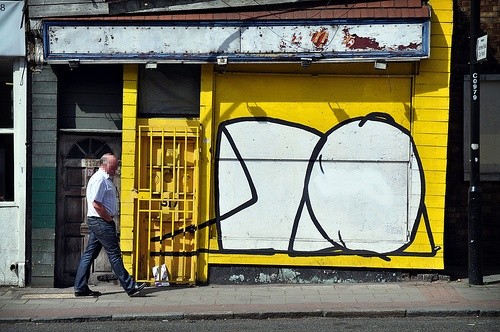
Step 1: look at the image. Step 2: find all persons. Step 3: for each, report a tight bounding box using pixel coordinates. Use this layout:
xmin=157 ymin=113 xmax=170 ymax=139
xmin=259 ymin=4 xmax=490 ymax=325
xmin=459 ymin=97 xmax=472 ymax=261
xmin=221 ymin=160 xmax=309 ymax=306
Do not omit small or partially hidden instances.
xmin=74 ymin=154 xmax=147 ymax=299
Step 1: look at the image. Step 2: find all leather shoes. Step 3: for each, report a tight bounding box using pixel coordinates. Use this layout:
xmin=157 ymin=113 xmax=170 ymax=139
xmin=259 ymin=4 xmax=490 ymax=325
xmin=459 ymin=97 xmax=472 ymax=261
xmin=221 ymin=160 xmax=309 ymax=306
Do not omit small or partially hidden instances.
xmin=75 ymin=287 xmax=102 ymax=297
xmin=129 ymin=281 xmax=147 ymax=297
